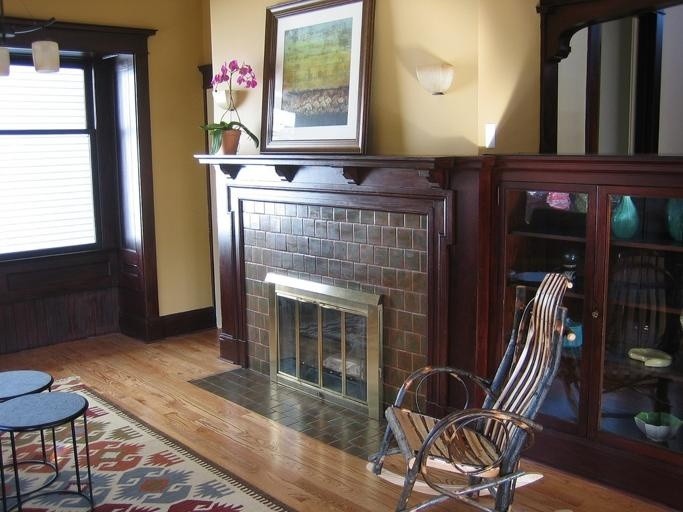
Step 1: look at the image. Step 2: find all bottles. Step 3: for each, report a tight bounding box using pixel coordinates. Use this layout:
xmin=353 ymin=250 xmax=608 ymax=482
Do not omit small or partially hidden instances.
xmin=610 ymin=196 xmax=639 ymax=240
xmin=562 ymin=253 xmax=578 ymax=292
xmin=665 ymin=199 xmax=683 ymax=242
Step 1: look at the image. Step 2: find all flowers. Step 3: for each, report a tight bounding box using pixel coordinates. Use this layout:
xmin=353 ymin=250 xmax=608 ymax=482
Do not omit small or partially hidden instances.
xmin=200 ymin=60 xmax=259 ymax=153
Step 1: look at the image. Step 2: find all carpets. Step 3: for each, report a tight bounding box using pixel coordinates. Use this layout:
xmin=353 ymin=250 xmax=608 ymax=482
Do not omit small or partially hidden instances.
xmin=0 ymin=377 xmax=301 ymax=512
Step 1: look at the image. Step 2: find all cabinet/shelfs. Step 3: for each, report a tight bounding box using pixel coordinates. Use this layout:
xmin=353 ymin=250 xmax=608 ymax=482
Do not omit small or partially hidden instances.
xmin=476 ymin=159 xmax=683 ymax=512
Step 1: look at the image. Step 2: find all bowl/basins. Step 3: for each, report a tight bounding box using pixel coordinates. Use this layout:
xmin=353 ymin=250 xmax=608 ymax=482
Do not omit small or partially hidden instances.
xmin=633 ymin=411 xmax=683 ymax=443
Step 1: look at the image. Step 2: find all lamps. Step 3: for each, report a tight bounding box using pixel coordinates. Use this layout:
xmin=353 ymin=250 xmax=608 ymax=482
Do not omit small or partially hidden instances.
xmin=213 ymin=90 xmax=246 ymax=110
xmin=417 ymin=64 xmax=454 ymax=95
xmin=0 ymin=0 xmax=59 ymax=77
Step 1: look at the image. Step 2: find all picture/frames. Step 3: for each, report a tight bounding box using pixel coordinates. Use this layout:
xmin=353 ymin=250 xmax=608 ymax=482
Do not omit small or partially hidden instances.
xmin=259 ymin=0 xmax=376 ymax=155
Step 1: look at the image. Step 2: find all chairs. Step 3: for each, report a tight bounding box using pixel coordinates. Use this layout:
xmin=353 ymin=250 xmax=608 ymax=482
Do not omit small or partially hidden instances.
xmin=367 ymin=272 xmax=571 ymax=511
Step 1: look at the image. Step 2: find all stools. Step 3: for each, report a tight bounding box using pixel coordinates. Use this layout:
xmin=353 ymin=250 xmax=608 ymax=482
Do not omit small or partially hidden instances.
xmin=0 ymin=369 xmax=59 ymax=499
xmin=0 ymin=392 xmax=93 ymax=512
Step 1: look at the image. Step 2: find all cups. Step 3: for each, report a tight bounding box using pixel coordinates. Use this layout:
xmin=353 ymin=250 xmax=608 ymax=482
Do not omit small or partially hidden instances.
xmin=563 ymin=322 xmax=583 ymax=349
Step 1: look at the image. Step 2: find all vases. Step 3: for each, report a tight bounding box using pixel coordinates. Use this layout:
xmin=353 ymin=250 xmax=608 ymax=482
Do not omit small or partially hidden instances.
xmin=222 ymin=130 xmax=241 ymax=155
